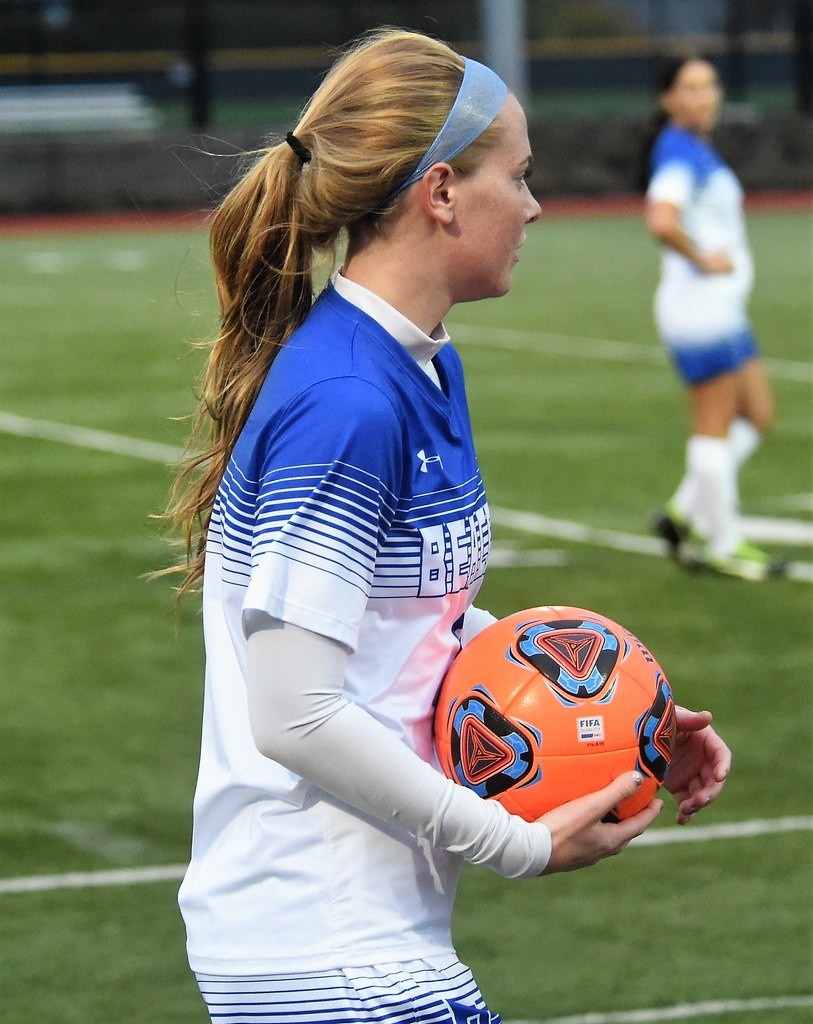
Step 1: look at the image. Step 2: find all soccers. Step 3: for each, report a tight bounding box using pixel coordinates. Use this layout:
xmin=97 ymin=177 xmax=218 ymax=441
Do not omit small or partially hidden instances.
xmin=432 ymin=603 xmax=676 ymax=827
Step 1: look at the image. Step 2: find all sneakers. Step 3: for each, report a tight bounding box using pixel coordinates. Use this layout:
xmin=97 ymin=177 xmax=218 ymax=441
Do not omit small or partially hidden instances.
xmin=657 ymin=502 xmax=785 ymax=583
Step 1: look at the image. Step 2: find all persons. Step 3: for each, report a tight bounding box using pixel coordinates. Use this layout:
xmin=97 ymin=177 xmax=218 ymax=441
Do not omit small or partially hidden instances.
xmin=647 ymin=57 xmax=785 ymax=587
xmin=142 ymin=25 xmax=733 ymax=1023
